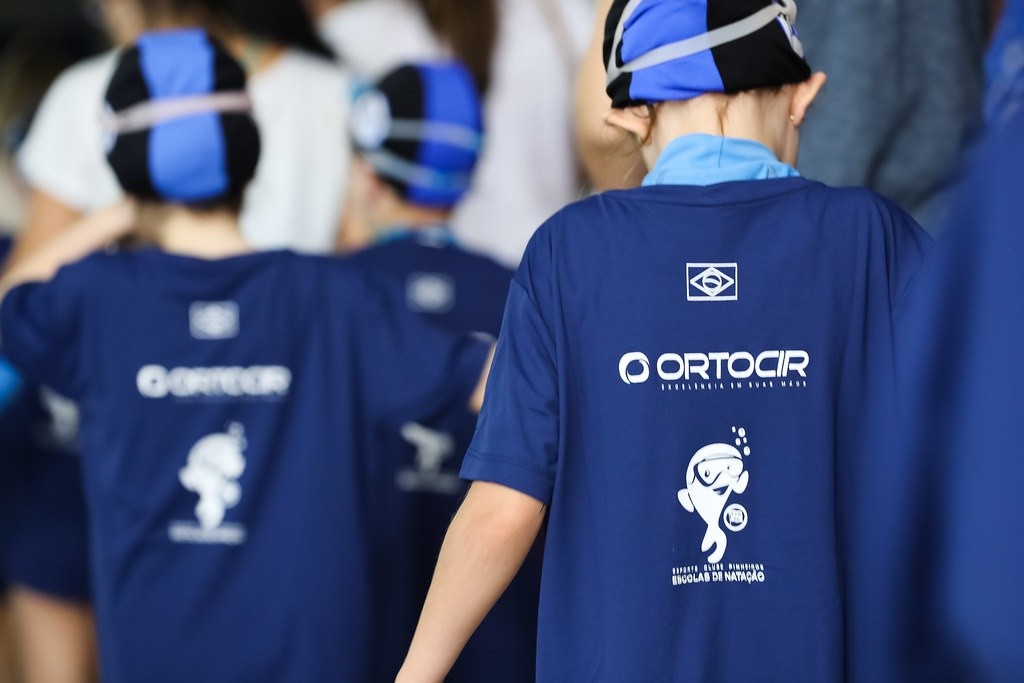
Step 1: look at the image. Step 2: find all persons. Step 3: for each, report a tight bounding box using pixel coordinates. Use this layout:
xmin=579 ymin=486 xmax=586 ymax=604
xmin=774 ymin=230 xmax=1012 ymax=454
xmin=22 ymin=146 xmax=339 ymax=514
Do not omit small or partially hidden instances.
xmin=395 ymin=0 xmax=939 ymax=683
xmin=1 ymin=0 xmax=1024 ymax=683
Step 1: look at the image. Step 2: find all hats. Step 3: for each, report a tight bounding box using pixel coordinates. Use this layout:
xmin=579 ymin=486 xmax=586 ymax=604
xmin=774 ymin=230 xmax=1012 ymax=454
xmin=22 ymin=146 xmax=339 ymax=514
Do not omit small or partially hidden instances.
xmin=602 ymin=0 xmax=811 ymax=109
xmin=106 ymin=29 xmax=262 ymax=203
xmin=346 ymin=62 xmax=484 ymax=206
